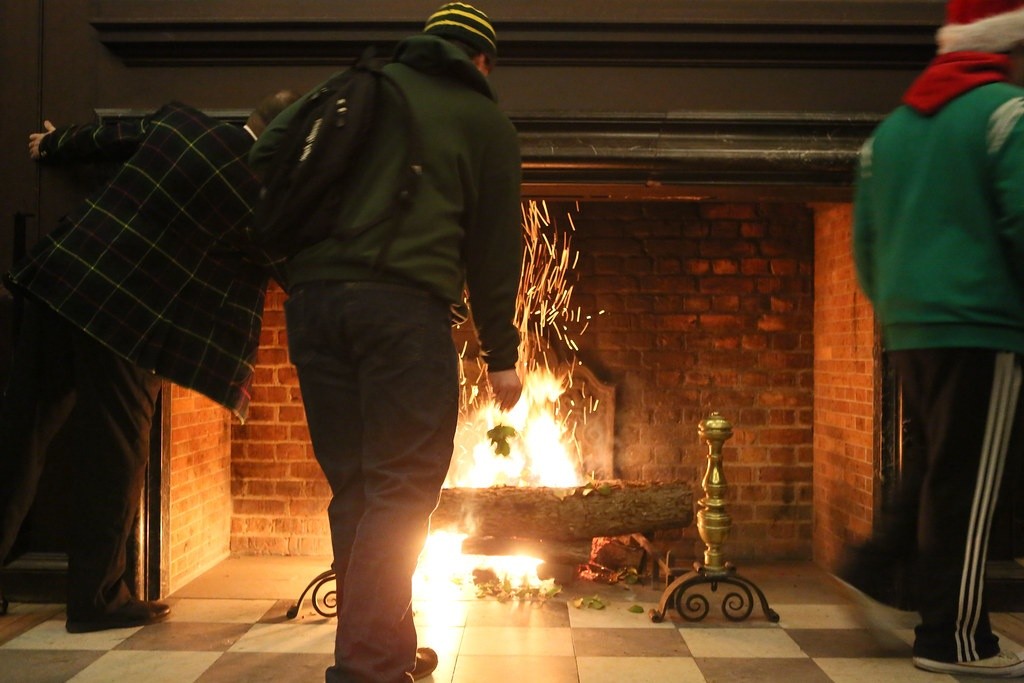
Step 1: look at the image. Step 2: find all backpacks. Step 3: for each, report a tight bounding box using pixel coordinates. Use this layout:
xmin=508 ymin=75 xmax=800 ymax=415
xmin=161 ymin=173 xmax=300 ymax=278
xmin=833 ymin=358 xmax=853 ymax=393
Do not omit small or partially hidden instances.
xmin=243 ymin=59 xmax=422 ymax=258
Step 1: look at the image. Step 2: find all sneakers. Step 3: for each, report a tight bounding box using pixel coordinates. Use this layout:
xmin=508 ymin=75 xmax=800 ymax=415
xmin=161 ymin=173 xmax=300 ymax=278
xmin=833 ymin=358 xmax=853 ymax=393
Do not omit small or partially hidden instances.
xmin=912 ymin=652 xmax=1024 ymax=677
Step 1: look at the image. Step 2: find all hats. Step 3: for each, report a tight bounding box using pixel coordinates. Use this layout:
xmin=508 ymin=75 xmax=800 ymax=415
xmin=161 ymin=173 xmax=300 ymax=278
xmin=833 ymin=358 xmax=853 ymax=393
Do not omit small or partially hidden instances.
xmin=933 ymin=1 xmax=1023 ymax=56
xmin=423 ymin=2 xmax=496 ymax=74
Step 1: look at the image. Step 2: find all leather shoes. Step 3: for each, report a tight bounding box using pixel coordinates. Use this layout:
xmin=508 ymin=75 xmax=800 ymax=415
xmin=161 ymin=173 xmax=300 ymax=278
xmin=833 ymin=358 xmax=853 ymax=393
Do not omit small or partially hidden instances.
xmin=65 ymin=597 xmax=172 ymax=633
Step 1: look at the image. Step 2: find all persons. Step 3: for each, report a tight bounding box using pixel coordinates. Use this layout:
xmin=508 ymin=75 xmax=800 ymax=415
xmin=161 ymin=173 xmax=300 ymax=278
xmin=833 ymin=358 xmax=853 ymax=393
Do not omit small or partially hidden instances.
xmin=828 ymin=0 xmax=1024 ymax=683
xmin=254 ymin=4 xmax=522 ymax=683
xmin=1 ymin=87 xmax=303 ymax=633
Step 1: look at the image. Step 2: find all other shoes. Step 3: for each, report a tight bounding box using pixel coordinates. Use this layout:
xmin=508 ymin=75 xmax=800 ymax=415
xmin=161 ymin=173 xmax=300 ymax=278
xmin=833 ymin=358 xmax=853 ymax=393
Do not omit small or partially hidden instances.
xmin=412 ymin=647 xmax=438 ymax=679
